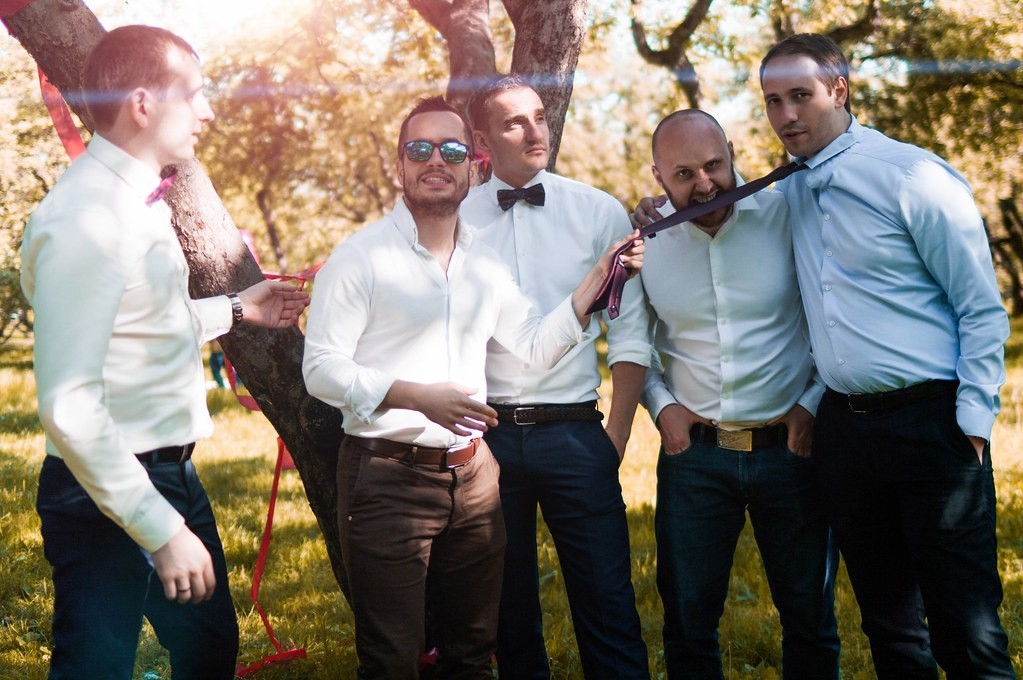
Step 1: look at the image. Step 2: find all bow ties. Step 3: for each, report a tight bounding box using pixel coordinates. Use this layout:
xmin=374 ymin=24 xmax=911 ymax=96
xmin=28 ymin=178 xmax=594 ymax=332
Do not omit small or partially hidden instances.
xmin=497 ymin=182 xmax=546 ymax=212
xmin=146 ymin=174 xmax=177 ymax=205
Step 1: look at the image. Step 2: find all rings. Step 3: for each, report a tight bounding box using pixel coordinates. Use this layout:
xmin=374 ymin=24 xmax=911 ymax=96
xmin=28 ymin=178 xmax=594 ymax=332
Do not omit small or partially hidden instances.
xmin=178 ymin=587 xmax=191 ymax=592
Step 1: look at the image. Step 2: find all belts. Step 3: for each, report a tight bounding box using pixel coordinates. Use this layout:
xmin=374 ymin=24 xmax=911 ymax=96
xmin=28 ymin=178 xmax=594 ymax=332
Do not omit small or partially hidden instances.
xmin=656 ymin=414 xmax=789 ymax=452
xmin=492 ymin=406 xmax=604 ymax=425
xmin=823 ymin=381 xmax=955 ymax=414
xmin=134 ymin=441 xmax=195 ymax=462
xmin=345 ymin=433 xmax=480 ymax=469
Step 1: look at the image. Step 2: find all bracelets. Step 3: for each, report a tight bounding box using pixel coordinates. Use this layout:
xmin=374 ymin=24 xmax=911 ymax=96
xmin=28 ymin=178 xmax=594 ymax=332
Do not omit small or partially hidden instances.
xmin=226 ymin=292 xmax=243 ymax=326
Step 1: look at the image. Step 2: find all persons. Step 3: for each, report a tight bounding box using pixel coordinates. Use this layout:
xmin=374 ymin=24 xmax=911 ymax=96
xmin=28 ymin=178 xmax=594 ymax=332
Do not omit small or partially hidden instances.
xmin=303 ymin=96 xmax=645 ymax=680
xmin=631 ymin=33 xmax=1015 ymax=680
xmin=20 ymin=25 xmax=312 ymax=680
xmin=459 ymin=75 xmax=651 ymax=680
xmin=638 ymin=109 xmax=841 ymax=680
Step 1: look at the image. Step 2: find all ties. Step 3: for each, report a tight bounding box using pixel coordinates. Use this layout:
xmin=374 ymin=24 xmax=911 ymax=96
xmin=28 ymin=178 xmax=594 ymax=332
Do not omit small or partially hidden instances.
xmin=584 ymin=156 xmax=810 ymax=321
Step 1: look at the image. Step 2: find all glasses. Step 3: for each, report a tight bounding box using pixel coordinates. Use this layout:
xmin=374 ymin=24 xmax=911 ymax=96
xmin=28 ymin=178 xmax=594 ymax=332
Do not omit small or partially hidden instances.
xmin=399 ymin=140 xmax=473 ymax=164
